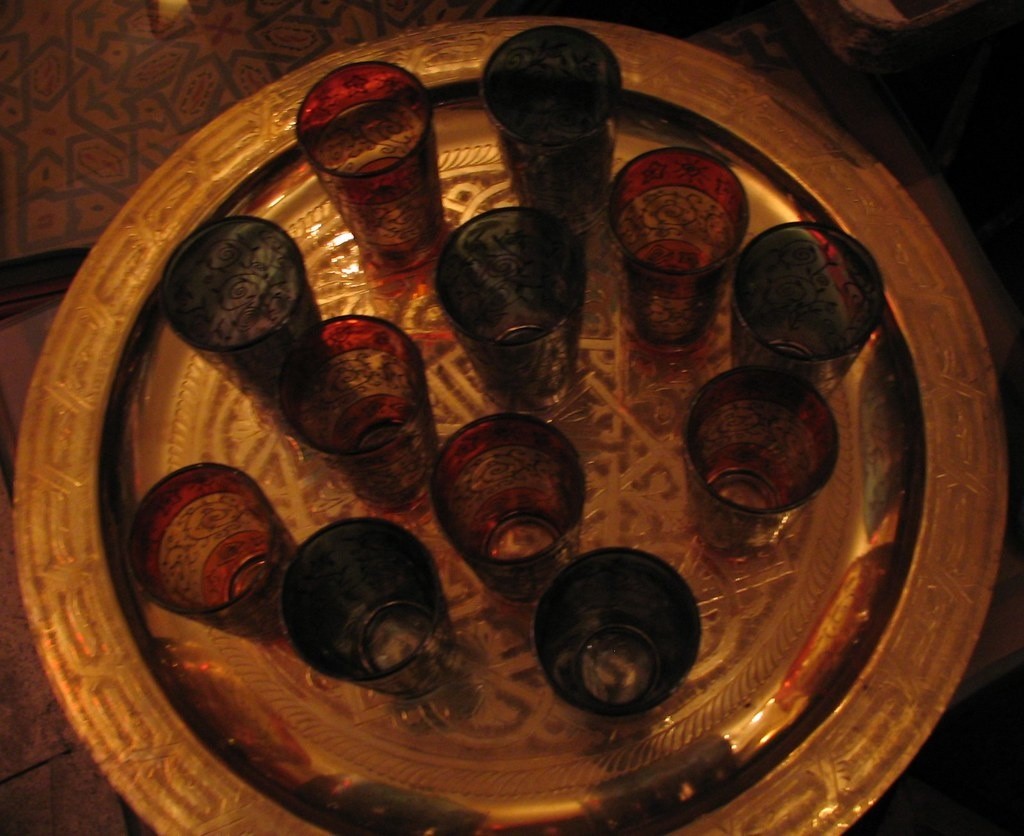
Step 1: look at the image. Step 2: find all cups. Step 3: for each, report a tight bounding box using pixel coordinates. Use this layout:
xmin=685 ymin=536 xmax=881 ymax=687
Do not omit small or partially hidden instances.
xmin=123 ymin=24 xmax=886 ymax=717
